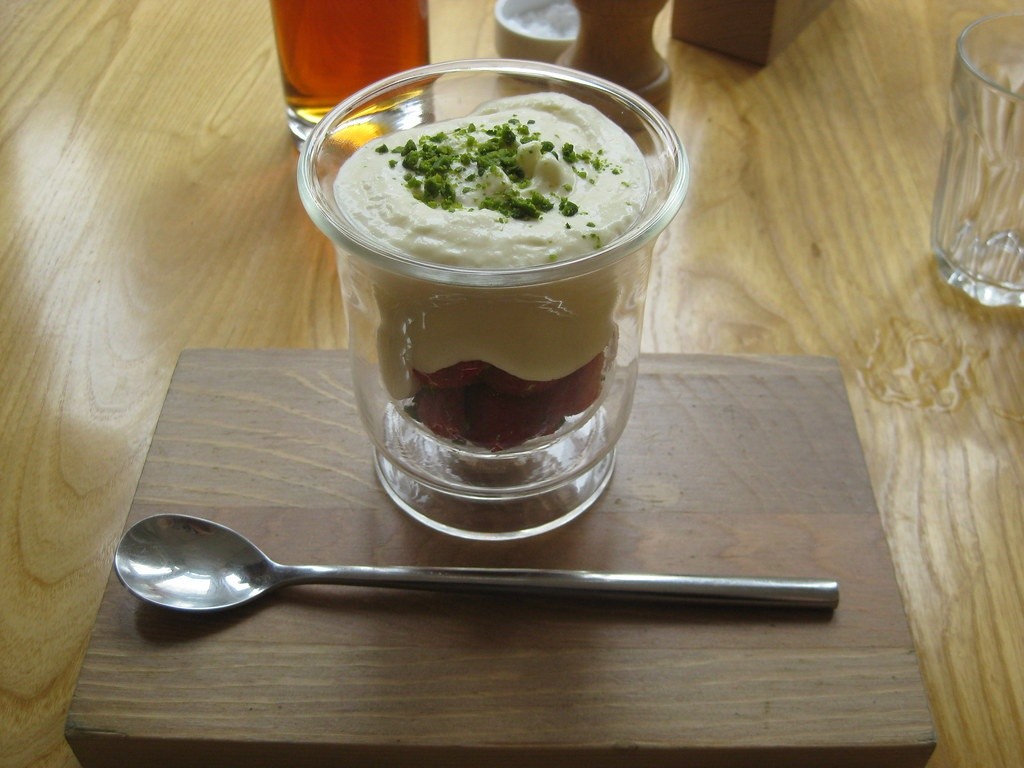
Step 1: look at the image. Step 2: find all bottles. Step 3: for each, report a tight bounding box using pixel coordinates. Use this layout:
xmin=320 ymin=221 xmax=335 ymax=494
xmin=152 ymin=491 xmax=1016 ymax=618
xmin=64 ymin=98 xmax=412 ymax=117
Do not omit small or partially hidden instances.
xmin=546 ymin=0 xmax=671 ymax=111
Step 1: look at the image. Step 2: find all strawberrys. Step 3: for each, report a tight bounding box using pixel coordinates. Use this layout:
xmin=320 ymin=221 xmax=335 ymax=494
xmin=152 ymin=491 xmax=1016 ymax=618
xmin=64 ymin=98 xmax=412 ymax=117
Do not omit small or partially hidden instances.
xmin=408 ymin=344 xmax=607 ymax=452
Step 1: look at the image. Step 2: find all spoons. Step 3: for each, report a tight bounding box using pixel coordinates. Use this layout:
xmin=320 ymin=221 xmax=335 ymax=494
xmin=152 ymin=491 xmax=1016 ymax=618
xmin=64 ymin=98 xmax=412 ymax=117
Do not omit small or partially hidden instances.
xmin=113 ymin=515 xmax=841 ymax=610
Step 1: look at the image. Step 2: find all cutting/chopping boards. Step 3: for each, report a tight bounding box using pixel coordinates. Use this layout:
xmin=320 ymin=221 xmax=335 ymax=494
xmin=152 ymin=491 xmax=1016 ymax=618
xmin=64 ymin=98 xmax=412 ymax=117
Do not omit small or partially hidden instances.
xmin=64 ymin=347 xmax=939 ymax=767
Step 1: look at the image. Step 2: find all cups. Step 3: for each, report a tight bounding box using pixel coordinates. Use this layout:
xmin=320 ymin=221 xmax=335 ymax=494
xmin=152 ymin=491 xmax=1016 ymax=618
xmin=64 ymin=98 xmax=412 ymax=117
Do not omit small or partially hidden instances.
xmin=929 ymin=10 xmax=1024 ymax=308
xmin=298 ymin=60 xmax=689 ymax=542
xmin=271 ymin=0 xmax=430 ymax=145
xmin=495 ymin=0 xmax=580 ymax=64
